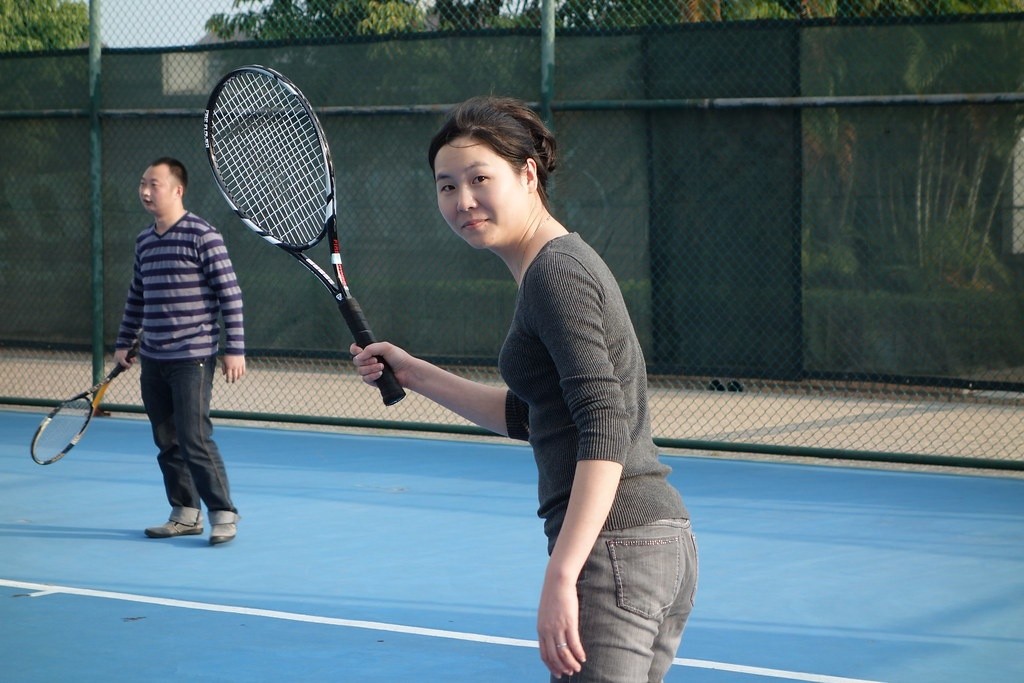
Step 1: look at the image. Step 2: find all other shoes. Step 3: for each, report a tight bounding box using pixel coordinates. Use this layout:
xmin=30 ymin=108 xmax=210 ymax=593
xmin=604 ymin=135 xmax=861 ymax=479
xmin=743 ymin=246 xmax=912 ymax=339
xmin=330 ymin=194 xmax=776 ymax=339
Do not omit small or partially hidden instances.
xmin=144 ymin=520 xmax=204 ymax=537
xmin=209 ymin=522 xmax=237 ymax=543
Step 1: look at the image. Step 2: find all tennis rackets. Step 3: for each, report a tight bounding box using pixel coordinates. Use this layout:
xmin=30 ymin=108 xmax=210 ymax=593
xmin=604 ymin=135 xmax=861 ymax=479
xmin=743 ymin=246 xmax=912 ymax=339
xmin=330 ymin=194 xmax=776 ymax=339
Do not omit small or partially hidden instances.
xmin=200 ymin=62 xmax=408 ymax=409
xmin=30 ymin=341 xmax=140 ymax=466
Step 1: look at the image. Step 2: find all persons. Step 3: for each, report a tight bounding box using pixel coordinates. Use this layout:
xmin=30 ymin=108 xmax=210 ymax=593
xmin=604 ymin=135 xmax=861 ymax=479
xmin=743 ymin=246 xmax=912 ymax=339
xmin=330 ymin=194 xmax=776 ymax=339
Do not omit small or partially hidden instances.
xmin=349 ymin=96 xmax=698 ymax=683
xmin=113 ymin=158 xmax=246 ymax=544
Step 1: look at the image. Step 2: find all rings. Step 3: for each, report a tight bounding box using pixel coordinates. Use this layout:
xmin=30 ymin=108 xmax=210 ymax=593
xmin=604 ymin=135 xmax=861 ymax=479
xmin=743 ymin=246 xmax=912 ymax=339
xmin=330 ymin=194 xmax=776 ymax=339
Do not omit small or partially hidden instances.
xmin=556 ymin=643 xmax=568 ymax=647
